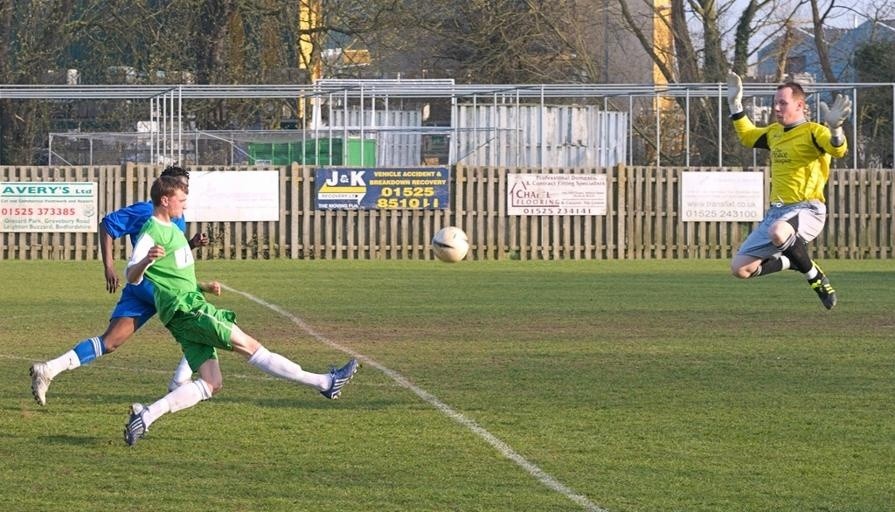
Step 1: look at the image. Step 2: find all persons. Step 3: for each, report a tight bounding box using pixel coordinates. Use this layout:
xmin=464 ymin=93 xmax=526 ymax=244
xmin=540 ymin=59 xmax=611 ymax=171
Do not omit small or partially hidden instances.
xmin=725 ymin=68 xmax=853 ymax=313
xmin=121 ymin=175 xmax=361 ymax=446
xmin=28 ymin=166 xmax=210 ymax=408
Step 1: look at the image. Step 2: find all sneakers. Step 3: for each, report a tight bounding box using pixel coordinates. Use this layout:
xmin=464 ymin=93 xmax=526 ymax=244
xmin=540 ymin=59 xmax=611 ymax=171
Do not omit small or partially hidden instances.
xmin=122 ymin=401 xmax=154 ymax=446
xmin=804 ymin=258 xmax=839 ymax=310
xmin=167 ymin=377 xmax=195 ymax=392
xmin=319 ymin=357 xmax=361 ymax=400
xmin=29 ymin=360 xmax=55 ymax=409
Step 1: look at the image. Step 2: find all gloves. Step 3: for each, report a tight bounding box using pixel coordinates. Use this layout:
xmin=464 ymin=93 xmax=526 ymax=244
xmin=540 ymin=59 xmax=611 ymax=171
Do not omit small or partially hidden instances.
xmin=725 ymin=68 xmax=746 ymax=116
xmin=819 ymin=92 xmax=854 ymax=137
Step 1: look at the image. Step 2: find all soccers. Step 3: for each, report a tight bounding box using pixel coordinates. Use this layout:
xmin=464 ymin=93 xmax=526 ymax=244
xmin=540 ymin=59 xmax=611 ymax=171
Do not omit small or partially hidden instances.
xmin=432 ymin=227 xmax=468 ymax=263
